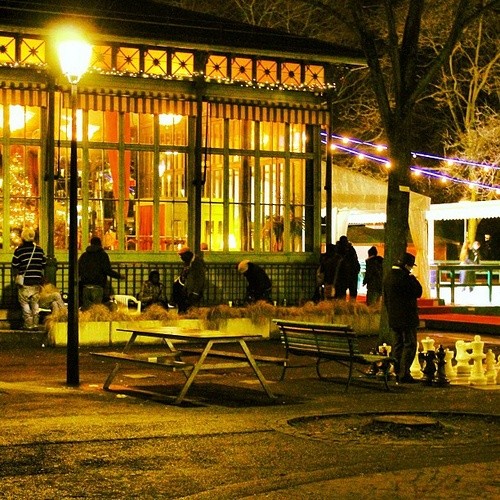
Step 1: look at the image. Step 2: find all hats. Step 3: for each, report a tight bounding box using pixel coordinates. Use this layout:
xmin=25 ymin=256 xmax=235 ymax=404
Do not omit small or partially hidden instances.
xmin=398 ymin=252 xmax=418 ymax=267
xmin=368 ymin=246 xmax=378 ymax=254
xmin=237 ymin=259 xmax=250 ymax=273
xmin=178 ymin=247 xmax=192 ymax=255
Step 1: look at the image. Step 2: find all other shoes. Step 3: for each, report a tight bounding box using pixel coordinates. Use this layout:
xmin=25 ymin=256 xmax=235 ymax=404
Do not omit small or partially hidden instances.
xmin=19 ymin=324 xmax=39 ymax=330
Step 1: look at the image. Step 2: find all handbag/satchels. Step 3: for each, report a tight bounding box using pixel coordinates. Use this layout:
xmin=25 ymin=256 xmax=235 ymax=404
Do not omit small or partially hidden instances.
xmin=15 ymin=274 xmax=24 ymax=286
xmin=324 ymin=282 xmax=335 ymax=298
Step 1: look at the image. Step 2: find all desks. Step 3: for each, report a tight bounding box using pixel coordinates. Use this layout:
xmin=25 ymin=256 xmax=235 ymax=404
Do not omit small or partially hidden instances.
xmin=436 ymin=264 xmax=500 ymax=304
xmin=87 ymin=328 xmax=291 ymax=407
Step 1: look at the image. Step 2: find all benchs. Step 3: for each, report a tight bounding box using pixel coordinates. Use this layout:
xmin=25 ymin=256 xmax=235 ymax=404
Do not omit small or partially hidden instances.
xmin=273 ymin=316 xmax=393 ymax=392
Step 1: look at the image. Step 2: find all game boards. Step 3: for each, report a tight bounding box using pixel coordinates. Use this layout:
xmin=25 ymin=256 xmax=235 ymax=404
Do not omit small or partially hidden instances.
xmin=364 ymin=363 xmax=500 ymax=391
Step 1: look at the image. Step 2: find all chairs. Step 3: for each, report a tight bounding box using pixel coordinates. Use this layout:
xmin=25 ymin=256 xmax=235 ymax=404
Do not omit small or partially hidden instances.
xmin=107 ymin=294 xmax=142 ymax=316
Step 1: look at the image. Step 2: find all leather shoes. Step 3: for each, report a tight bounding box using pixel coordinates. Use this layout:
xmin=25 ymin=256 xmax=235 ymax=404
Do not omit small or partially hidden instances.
xmin=396 ymin=372 xmax=421 ymax=384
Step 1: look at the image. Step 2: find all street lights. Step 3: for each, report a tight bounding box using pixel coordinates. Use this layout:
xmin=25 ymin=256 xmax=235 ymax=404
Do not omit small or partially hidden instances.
xmin=55 ymin=18 xmax=97 ymax=390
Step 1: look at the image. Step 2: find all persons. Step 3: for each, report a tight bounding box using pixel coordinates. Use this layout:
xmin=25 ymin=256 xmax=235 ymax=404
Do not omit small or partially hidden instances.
xmin=167 ymin=248 xmax=206 ymax=315
xmin=383 ymin=252 xmax=423 ymax=384
xmin=310 ymin=235 xmax=362 ymax=303
xmin=481 ymin=233 xmax=498 ymax=280
xmin=238 ymin=260 xmax=275 ymax=306
xmin=362 ymin=246 xmax=384 ymax=306
xmin=138 ymin=271 xmax=169 ymax=313
xmin=77 ymin=236 xmax=125 ymax=312
xmin=462 ymin=241 xmax=480 ymax=292
xmin=11 ymin=227 xmax=49 ymax=331
